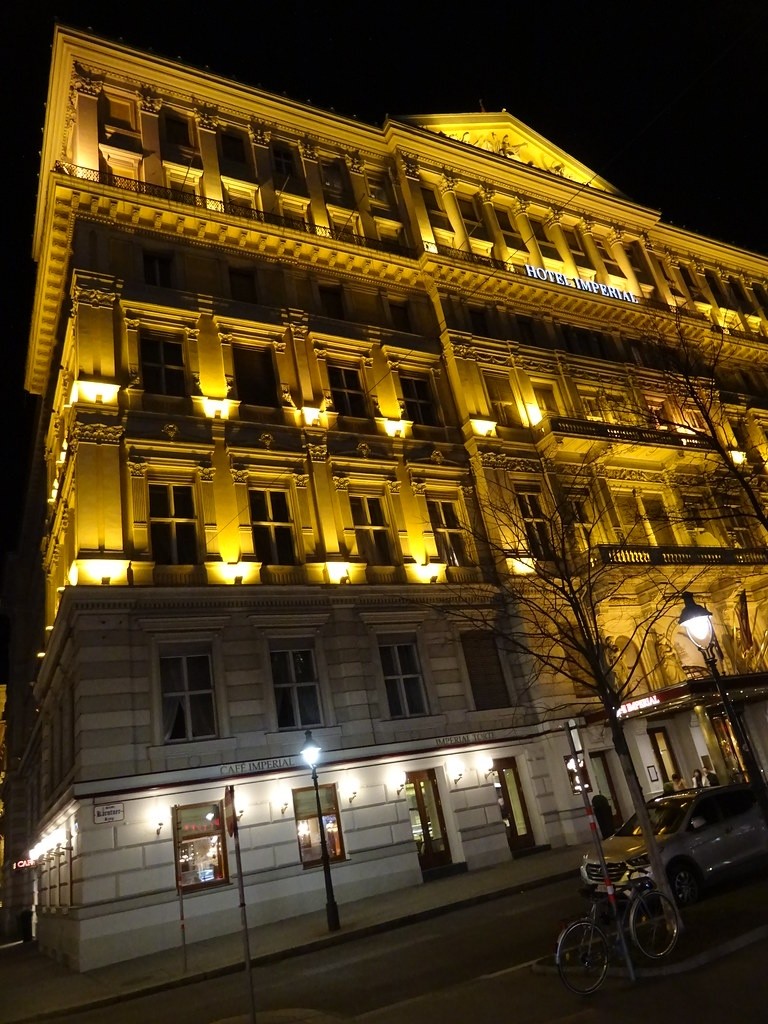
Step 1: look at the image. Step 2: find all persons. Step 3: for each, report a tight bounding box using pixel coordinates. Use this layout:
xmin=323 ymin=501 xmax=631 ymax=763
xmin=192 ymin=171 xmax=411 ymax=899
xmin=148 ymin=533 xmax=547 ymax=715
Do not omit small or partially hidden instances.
xmin=664 ymin=767 xmax=720 ymax=795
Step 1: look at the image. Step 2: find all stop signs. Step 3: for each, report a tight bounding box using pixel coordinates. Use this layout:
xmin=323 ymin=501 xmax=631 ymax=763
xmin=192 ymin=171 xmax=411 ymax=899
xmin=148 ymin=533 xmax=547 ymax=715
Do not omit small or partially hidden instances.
xmin=225 ymin=787 xmax=236 ymax=838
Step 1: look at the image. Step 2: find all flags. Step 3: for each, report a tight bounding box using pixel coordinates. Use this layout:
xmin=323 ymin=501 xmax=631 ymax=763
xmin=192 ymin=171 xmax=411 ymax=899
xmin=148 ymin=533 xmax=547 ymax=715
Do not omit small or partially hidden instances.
xmin=735 ymin=589 xmax=754 ymax=650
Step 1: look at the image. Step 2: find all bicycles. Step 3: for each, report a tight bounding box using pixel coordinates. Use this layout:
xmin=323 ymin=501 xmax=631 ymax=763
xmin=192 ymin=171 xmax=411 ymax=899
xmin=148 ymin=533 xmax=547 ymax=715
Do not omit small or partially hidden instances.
xmin=553 ymin=867 xmax=680 ymax=995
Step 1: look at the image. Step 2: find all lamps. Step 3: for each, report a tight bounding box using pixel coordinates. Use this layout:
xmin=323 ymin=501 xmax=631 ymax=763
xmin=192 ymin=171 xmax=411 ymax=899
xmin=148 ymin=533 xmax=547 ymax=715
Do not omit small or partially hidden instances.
xmin=29 ymin=829 xmax=73 ymax=868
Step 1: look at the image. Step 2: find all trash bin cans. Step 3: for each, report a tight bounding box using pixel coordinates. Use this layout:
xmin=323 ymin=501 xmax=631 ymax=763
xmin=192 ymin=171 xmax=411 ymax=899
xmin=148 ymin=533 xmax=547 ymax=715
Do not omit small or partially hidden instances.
xmin=16 ymin=910 xmax=33 ymax=943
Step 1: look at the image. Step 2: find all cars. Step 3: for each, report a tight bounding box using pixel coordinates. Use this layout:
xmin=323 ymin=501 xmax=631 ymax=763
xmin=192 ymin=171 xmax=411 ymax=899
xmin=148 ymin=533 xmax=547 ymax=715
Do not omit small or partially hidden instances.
xmin=581 ymin=783 xmax=767 ymax=906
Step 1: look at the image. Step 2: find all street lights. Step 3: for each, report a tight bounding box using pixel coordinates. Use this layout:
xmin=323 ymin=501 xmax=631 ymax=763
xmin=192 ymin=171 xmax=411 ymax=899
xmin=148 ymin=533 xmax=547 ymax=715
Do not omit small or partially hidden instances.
xmin=679 ymin=589 xmax=768 ymax=820
xmin=299 ymin=729 xmax=340 ymax=931
